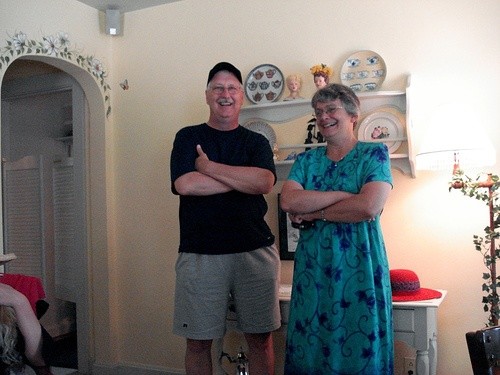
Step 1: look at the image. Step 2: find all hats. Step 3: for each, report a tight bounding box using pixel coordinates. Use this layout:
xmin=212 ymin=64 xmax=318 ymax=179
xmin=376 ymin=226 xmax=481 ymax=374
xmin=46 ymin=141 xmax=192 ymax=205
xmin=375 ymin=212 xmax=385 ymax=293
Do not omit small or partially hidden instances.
xmin=207 ymin=62 xmax=242 ymax=83
xmin=390 ymin=269 xmax=442 ymax=302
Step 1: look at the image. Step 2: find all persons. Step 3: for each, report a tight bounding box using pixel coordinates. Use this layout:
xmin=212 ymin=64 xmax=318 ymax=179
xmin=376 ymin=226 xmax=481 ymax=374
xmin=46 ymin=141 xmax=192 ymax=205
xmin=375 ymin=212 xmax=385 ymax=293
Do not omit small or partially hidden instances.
xmin=310 ymin=64 xmax=333 ymax=91
xmin=168 ymin=62 xmax=283 ymax=375
xmin=277 ymin=83 xmax=395 ymax=375
xmin=283 ymin=74 xmax=306 ymax=101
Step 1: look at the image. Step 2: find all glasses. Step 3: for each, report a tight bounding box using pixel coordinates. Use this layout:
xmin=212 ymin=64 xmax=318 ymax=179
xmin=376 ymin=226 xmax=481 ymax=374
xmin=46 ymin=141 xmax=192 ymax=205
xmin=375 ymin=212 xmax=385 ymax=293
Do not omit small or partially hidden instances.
xmin=312 ymin=105 xmax=345 ymax=117
xmin=207 ymin=85 xmax=242 ymax=94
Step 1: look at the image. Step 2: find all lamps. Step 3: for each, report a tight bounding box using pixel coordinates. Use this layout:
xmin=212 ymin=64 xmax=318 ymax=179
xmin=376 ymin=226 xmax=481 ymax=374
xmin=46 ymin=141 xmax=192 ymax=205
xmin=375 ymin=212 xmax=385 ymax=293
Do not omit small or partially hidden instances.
xmin=104 ymin=7 xmax=120 ymax=35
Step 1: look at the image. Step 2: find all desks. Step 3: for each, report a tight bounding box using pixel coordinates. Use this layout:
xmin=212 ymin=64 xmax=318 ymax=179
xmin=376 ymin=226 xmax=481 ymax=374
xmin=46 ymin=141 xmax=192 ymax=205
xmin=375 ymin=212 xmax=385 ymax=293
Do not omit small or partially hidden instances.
xmin=231 ymin=284 xmax=447 ymax=375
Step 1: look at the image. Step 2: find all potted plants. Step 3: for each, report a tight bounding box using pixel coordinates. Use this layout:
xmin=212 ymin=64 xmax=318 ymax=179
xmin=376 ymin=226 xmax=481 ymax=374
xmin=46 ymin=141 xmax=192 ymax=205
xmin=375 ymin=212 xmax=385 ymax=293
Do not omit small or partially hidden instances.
xmin=447 ymin=162 xmax=500 ymax=375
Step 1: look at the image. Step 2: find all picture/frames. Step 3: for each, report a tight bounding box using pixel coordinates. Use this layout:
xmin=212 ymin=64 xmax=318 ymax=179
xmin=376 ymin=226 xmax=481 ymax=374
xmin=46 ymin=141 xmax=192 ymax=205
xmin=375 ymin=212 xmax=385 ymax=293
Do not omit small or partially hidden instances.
xmin=276 ymin=193 xmax=300 ymax=262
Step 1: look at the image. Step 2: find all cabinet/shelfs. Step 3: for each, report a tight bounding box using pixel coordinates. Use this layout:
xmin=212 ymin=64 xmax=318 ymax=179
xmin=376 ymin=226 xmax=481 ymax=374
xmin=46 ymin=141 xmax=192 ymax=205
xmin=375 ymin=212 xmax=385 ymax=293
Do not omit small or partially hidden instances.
xmin=240 ymin=91 xmax=408 ymax=167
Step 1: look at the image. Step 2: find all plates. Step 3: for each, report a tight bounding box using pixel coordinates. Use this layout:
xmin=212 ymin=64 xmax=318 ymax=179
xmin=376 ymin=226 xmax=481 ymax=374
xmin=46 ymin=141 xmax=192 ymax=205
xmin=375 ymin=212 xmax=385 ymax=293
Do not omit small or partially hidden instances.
xmin=242 ymin=120 xmax=276 ymax=152
xmin=243 ymin=63 xmax=285 ymax=104
xmin=357 ymin=111 xmax=404 ymax=154
xmin=339 ymin=50 xmax=387 ymax=93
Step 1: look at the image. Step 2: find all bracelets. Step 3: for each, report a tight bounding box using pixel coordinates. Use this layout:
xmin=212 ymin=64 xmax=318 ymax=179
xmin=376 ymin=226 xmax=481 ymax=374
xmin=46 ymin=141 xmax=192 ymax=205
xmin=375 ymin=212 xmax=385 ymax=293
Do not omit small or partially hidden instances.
xmin=320 ymin=209 xmax=326 ymax=222
